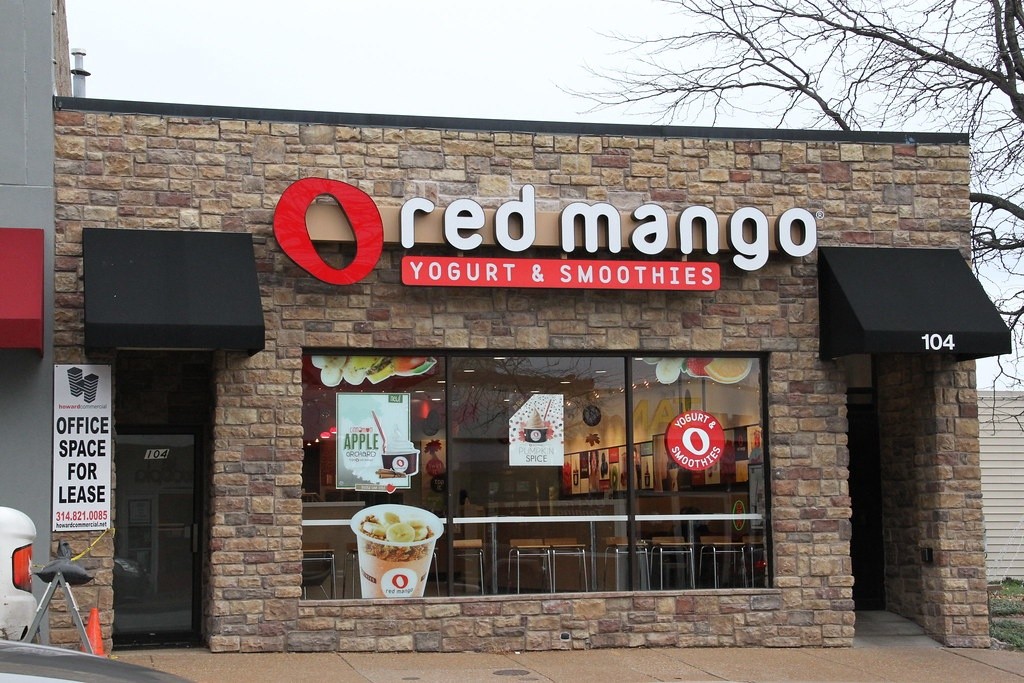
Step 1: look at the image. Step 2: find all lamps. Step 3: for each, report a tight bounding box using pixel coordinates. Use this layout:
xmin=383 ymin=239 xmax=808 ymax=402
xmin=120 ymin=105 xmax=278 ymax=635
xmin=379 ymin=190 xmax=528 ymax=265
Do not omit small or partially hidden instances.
xmin=320 ymin=431 xmax=331 ymax=439
xmin=328 ymin=426 xmax=336 ymax=434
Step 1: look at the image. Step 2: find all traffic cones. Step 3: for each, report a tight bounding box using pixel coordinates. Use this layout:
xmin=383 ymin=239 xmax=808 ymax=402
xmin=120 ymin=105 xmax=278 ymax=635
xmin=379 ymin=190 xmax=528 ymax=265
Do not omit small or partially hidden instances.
xmin=80 ymin=608 xmax=104 ymax=655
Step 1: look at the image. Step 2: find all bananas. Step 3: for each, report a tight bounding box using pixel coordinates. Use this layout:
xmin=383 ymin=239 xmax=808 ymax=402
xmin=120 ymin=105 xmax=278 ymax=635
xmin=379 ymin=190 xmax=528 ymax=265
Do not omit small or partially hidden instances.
xmin=363 ymin=513 xmax=429 ymax=543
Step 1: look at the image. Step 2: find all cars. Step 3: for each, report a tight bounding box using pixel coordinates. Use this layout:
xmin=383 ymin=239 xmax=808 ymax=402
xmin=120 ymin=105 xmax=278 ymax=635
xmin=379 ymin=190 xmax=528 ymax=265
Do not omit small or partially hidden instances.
xmin=1 ymin=506 xmax=39 ymax=642
xmin=0 ymin=638 xmax=196 ymax=683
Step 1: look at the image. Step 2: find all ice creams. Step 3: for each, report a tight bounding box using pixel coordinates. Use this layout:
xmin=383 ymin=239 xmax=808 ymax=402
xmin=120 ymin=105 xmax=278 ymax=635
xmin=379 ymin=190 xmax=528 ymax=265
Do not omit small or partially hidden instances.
xmin=523 ymin=409 xmax=548 ymax=443
xmin=381 ymin=430 xmax=420 ymax=477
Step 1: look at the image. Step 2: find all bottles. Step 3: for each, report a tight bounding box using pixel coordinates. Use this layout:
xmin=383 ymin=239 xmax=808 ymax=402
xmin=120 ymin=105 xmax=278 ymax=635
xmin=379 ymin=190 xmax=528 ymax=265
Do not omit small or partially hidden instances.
xmin=644 ymin=463 xmax=650 ymax=487
xmin=572 ymin=460 xmax=579 ymax=486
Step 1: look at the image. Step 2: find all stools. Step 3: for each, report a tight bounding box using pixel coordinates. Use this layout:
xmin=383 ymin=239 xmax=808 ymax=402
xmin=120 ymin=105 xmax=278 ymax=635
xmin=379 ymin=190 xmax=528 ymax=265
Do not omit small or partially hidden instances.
xmin=298 ymin=536 xmax=765 ymax=601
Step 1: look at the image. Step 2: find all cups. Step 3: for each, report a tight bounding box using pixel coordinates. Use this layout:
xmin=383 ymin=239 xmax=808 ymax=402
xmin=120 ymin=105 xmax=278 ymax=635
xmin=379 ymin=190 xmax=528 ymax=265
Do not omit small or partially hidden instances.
xmin=350 ymin=504 xmax=444 ymax=598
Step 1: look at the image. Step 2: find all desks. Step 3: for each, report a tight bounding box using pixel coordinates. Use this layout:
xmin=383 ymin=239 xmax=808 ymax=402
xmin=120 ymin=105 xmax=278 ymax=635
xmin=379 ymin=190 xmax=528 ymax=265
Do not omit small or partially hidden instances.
xmin=300 ymin=514 xmax=763 ymax=596
